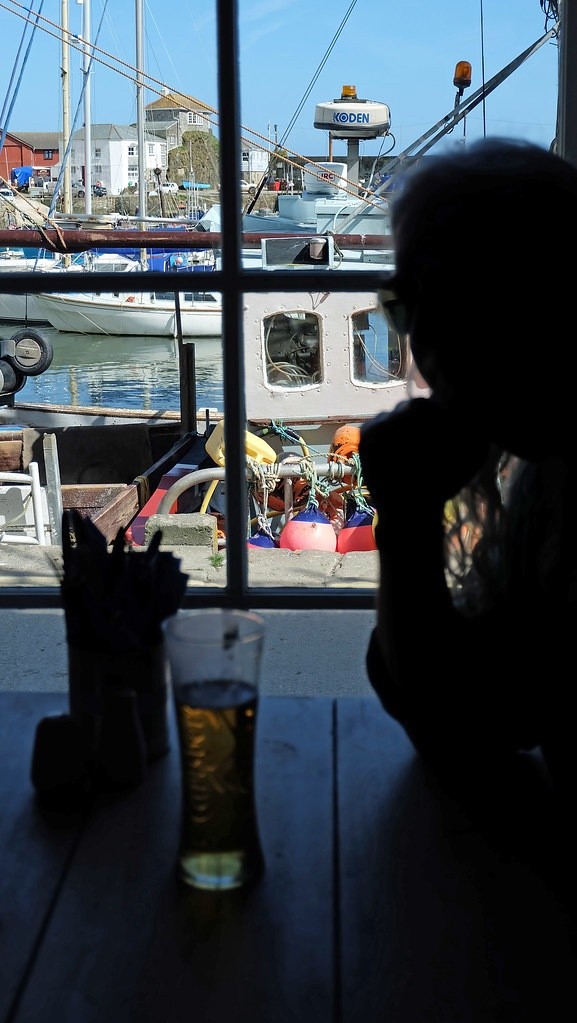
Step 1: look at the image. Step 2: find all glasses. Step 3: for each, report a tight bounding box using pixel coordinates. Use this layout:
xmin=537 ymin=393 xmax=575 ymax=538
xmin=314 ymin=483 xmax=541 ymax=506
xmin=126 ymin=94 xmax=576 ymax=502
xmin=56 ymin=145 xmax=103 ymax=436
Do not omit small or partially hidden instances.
xmin=377 ymin=275 xmax=408 ymax=334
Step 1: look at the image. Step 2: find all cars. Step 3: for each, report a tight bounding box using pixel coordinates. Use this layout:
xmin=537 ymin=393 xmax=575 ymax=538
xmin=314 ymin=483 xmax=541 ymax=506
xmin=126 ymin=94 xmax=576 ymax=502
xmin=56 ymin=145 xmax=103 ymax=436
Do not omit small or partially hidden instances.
xmin=91 ymin=185 xmax=107 ymax=197
xmin=0 ymin=189 xmax=14 ymax=200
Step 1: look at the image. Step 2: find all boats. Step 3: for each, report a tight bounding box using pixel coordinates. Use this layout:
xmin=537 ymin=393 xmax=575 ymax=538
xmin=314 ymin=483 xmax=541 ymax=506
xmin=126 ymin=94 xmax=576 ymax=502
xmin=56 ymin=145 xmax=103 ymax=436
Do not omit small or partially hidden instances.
xmin=182 ymin=179 xmax=210 ymax=189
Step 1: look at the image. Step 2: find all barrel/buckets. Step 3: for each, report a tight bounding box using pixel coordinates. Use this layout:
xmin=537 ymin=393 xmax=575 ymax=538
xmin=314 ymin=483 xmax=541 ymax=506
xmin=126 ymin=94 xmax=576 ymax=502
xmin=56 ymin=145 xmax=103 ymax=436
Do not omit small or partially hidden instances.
xmin=205 ymin=420 xmax=277 ymax=467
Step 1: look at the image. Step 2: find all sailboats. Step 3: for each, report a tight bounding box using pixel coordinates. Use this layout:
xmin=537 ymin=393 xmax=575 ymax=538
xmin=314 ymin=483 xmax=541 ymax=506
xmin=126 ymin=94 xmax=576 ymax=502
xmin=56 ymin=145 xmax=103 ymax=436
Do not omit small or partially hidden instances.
xmin=0 ymin=0 xmax=561 ymax=338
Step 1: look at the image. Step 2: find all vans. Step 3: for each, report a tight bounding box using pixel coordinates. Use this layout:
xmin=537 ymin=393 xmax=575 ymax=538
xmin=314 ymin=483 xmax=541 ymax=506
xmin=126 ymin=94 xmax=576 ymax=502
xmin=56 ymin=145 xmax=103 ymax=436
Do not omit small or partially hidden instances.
xmin=158 ymin=182 xmax=179 ymax=195
xmin=49 ymin=181 xmax=85 ymax=197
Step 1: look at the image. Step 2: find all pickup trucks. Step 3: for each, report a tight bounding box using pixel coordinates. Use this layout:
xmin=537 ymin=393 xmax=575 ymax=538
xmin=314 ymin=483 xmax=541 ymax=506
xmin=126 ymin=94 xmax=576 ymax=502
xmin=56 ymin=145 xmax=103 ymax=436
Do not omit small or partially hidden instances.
xmin=217 ymin=179 xmax=257 ymax=194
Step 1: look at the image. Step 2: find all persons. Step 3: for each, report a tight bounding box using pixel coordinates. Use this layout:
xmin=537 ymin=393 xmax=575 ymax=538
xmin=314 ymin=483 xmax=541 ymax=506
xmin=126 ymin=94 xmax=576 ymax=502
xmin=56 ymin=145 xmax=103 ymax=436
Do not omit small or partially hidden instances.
xmin=359 ymin=139 xmax=577 ymax=874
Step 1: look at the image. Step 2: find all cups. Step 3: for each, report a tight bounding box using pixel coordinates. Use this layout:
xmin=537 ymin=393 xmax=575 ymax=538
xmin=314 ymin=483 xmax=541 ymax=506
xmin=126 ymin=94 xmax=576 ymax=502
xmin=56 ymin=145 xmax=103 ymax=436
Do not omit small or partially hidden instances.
xmin=162 ymin=611 xmax=269 ymax=891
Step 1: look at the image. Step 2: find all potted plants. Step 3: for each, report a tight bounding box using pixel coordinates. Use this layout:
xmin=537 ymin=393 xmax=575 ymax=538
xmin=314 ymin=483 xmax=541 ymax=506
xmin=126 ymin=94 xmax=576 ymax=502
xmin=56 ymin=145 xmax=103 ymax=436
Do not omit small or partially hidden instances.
xmin=203 ymin=552 xmax=228 ymax=581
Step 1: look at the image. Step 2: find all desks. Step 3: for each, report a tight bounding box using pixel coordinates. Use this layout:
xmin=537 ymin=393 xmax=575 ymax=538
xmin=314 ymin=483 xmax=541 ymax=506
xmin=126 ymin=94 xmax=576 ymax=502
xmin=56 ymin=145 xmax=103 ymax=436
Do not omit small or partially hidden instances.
xmin=1 ymin=682 xmax=513 ymax=1023
